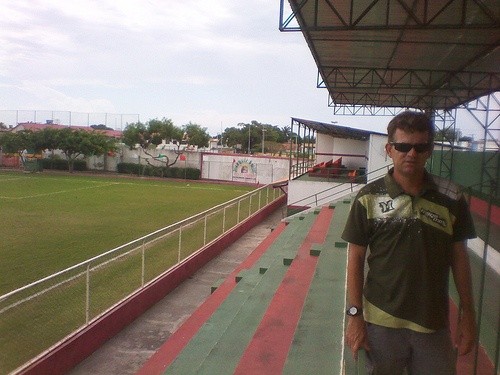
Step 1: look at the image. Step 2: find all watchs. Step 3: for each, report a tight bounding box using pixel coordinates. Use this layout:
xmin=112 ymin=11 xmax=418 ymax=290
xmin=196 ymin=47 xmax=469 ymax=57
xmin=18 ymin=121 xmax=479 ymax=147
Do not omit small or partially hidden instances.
xmin=347 ymin=306 xmax=363 ymax=316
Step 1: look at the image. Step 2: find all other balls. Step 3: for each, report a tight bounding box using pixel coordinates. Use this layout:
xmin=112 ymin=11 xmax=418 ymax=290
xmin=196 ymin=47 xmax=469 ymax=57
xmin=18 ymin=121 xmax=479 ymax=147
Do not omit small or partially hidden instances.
xmin=180 ymin=155 xmax=186 ymax=160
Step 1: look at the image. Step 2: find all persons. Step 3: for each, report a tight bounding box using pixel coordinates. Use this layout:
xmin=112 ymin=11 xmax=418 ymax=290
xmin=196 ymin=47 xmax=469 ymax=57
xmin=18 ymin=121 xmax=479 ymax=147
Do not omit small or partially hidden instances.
xmin=342 ymin=111 xmax=479 ymax=375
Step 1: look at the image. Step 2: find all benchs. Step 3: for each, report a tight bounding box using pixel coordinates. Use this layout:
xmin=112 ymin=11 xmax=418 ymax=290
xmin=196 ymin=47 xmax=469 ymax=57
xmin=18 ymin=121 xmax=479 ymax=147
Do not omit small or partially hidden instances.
xmin=308 ymin=156 xmax=342 ymax=173
